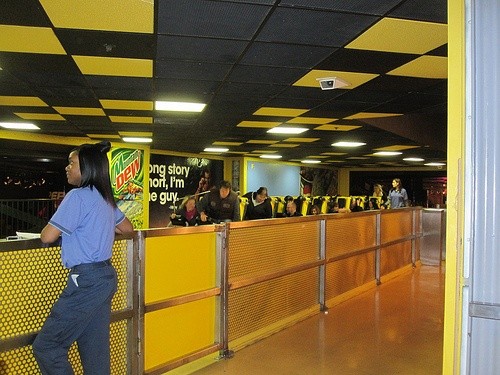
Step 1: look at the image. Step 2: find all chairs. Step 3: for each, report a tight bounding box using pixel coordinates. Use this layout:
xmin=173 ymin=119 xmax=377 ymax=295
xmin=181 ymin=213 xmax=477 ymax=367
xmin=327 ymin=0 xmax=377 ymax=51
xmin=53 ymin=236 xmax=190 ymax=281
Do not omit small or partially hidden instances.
xmin=167 ymin=193 xmax=419 ymax=227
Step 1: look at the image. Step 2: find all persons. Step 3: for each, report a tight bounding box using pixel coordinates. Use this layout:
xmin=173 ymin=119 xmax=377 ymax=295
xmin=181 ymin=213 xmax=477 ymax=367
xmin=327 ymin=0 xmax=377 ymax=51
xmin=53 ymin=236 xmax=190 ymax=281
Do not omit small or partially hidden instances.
xmin=32 ymin=143 xmax=133 ymax=375
xmin=168 ymin=177 xmax=409 ymax=226
xmin=196 ymin=168 xmax=215 ymax=194
xmin=34 ymin=206 xmax=52 ymax=220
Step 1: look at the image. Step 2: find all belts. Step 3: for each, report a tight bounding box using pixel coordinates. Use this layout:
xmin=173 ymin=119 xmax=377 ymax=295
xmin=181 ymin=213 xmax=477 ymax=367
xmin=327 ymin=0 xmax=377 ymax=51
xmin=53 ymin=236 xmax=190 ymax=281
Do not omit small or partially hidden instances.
xmin=70 ymin=260 xmax=112 ymax=272
